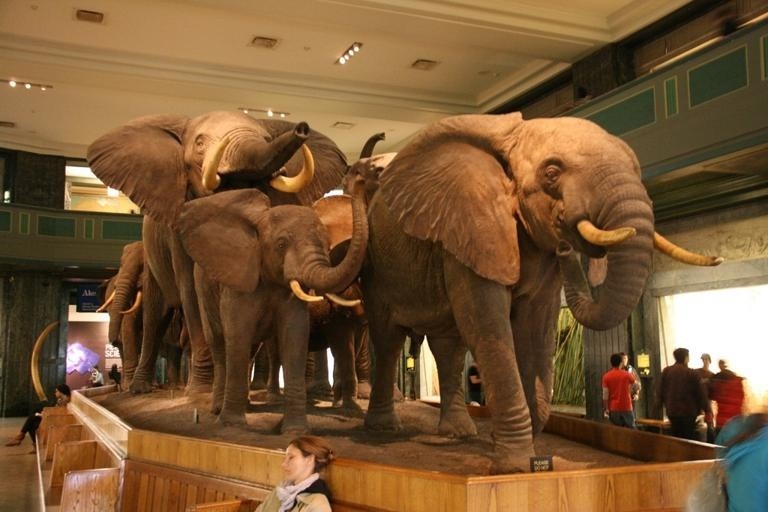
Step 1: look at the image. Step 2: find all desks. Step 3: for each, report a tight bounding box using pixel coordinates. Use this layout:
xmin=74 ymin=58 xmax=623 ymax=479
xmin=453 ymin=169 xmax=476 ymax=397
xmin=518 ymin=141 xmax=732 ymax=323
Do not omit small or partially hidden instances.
xmin=634 ymin=418 xmax=673 ymax=429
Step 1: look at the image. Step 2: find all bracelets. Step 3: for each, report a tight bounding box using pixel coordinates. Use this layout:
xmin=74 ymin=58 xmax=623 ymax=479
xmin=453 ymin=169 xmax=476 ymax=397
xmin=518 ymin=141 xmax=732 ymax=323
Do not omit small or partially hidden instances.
xmin=602 ymin=408 xmax=607 ymax=413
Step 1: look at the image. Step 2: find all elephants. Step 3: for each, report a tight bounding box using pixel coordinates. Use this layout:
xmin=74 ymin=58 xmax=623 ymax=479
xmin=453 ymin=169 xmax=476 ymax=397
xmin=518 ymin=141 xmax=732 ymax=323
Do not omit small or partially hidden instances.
xmin=95 ymin=237 xmax=184 ymax=393
xmin=175 ymin=159 xmax=374 ymax=436
xmin=84 ymin=110 xmax=350 ymax=394
xmin=362 ymin=109 xmax=724 ymax=476
xmin=300 ymin=126 xmax=419 ymax=405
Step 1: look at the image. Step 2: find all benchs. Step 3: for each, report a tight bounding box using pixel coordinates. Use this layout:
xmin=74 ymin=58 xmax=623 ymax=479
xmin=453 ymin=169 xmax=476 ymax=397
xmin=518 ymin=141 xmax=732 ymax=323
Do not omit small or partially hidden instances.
xmin=36 ymin=406 xmax=118 ymax=511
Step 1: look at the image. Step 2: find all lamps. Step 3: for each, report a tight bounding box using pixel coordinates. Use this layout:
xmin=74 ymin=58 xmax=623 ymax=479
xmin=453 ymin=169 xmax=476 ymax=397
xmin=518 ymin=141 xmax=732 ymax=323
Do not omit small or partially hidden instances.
xmin=238 ymin=107 xmax=291 ymax=119
xmin=405 ymin=357 xmax=416 ymax=371
xmin=1 ymin=78 xmax=53 ymax=94
xmin=637 ymin=351 xmax=651 ymax=376
xmin=334 ymin=41 xmax=362 ymax=66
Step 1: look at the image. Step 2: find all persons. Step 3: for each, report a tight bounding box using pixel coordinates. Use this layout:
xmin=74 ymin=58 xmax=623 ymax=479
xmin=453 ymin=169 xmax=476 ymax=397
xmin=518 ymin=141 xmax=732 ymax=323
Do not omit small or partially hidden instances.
xmin=695 ymin=354 xmax=715 ymax=442
xmin=708 ymin=360 xmax=745 ymax=440
xmin=601 ymin=354 xmax=638 ymax=429
xmin=655 ymin=348 xmax=714 ymax=444
xmin=4 ymin=383 xmax=71 ymax=453
xmin=467 ymin=361 xmax=484 ymax=406
xmin=620 ymin=351 xmax=641 ymax=422
xmin=256 ymin=435 xmax=333 ymax=512
xmin=89 ymin=365 xmax=103 ymax=385
xmin=107 ymin=363 xmax=121 ymax=384
